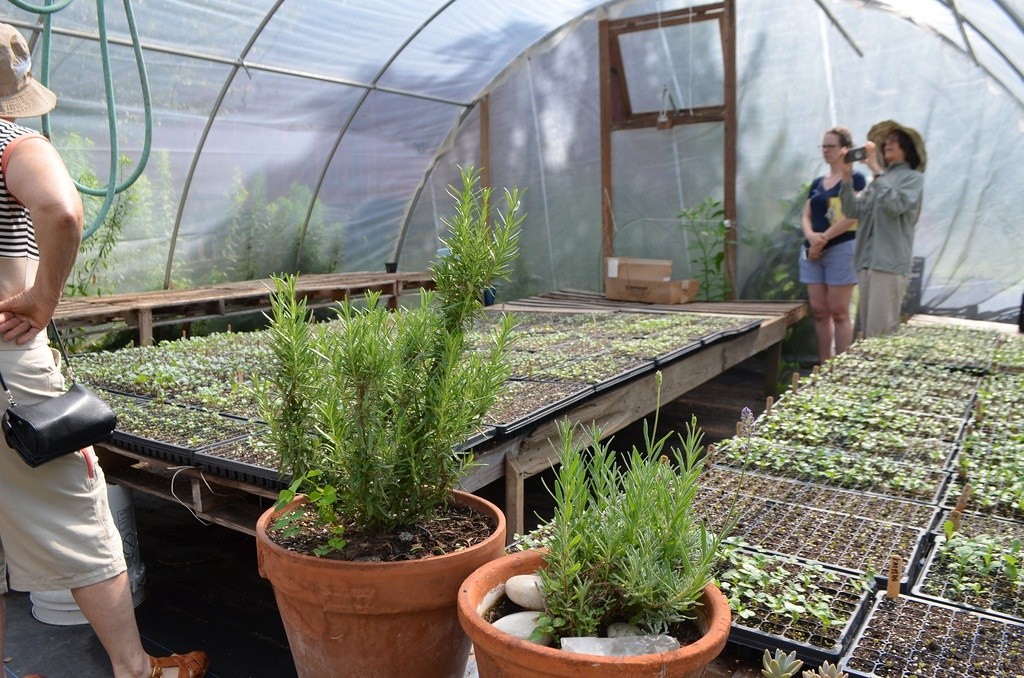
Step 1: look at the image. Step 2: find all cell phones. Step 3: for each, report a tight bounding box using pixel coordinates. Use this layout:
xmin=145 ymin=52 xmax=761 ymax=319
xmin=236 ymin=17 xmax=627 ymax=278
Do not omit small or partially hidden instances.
xmin=845 ymin=147 xmax=868 ymax=163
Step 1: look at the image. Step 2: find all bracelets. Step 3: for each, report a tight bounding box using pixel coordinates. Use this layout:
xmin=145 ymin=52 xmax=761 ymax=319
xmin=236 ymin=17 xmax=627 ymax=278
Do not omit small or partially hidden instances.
xmin=875 ymin=173 xmax=886 ymax=177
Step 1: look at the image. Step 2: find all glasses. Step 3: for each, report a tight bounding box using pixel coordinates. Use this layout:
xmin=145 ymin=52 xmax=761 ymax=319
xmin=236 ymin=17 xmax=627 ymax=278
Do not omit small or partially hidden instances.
xmin=883 ymin=137 xmax=900 ymax=144
xmin=817 ymin=144 xmax=842 ymax=149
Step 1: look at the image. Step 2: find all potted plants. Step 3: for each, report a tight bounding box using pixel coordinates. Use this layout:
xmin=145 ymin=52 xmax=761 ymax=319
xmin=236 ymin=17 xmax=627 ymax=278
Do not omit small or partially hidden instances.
xmin=256 ymin=159 xmax=524 ymax=678
xmin=460 ymin=369 xmax=737 ymax=678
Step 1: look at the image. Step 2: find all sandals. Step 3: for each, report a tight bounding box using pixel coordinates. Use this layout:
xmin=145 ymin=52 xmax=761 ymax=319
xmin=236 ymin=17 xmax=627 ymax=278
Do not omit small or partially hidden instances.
xmin=146 ymin=650 xmax=209 ymax=678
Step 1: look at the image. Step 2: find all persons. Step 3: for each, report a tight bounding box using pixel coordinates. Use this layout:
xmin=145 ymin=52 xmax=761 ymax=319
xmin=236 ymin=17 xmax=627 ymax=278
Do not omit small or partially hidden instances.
xmin=1 ymin=24 xmax=210 ymax=678
xmin=840 ymin=120 xmax=926 ymax=338
xmin=798 ymin=126 xmax=866 ymax=362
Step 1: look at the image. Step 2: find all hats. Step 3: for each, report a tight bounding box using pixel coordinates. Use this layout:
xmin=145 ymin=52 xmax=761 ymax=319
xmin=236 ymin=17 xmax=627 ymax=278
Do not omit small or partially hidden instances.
xmin=0 ymin=22 xmax=57 ymax=118
xmin=867 ymin=119 xmax=928 ymax=174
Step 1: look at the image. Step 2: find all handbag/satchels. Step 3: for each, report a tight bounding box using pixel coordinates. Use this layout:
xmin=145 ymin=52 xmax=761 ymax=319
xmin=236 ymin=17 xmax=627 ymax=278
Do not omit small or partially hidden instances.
xmin=1 ymin=382 xmax=117 ymax=469
